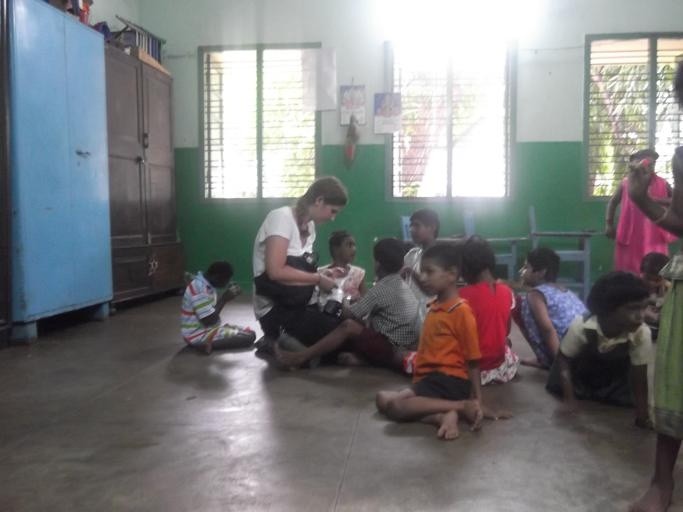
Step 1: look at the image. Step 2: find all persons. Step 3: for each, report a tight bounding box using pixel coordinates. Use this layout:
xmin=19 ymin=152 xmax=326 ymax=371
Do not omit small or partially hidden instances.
xmin=180 ymin=260 xmax=257 ymax=352
xmin=309 ymin=230 xmax=371 ymax=317
xmin=252 ymin=174 xmax=368 ymax=371
xmin=403 ymin=210 xmax=457 ymax=320
xmin=639 ymin=253 xmax=674 ymax=344
xmin=375 ymin=240 xmax=515 ymax=441
xmin=604 ymin=147 xmax=674 ymax=284
xmin=275 ymin=237 xmax=426 ymax=377
xmin=624 ymin=55 xmax=681 ymax=511
xmin=543 ymin=270 xmax=656 ymax=436
xmin=508 ymin=244 xmax=594 ymax=373
xmin=456 ymin=235 xmax=522 ymax=388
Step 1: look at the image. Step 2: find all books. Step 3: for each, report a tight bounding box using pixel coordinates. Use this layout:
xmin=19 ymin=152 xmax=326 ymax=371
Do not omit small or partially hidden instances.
xmin=109 ymin=27 xmax=166 ymax=64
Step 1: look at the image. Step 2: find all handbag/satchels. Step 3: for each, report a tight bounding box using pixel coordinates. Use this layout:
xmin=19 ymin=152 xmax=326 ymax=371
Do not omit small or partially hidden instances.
xmin=253 ymin=252 xmax=319 ymax=308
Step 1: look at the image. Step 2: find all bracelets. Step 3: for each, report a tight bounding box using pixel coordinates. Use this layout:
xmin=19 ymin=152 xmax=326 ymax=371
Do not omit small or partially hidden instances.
xmin=315 ymin=273 xmax=322 ymax=286
xmin=648 ymin=203 xmax=671 ymax=226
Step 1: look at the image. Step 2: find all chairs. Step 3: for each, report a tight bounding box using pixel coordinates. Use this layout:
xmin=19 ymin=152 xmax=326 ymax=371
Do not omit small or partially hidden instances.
xmin=527 ymin=205 xmax=601 ymax=308
xmin=461 ymin=209 xmax=522 ymax=281
xmin=399 ymin=214 xmax=415 ymax=242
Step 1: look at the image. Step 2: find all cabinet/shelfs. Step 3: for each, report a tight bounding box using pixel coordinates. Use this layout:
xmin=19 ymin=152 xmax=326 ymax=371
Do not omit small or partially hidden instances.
xmin=1 ymin=0 xmax=112 ymax=343
xmin=106 ymin=43 xmax=185 ymax=312
